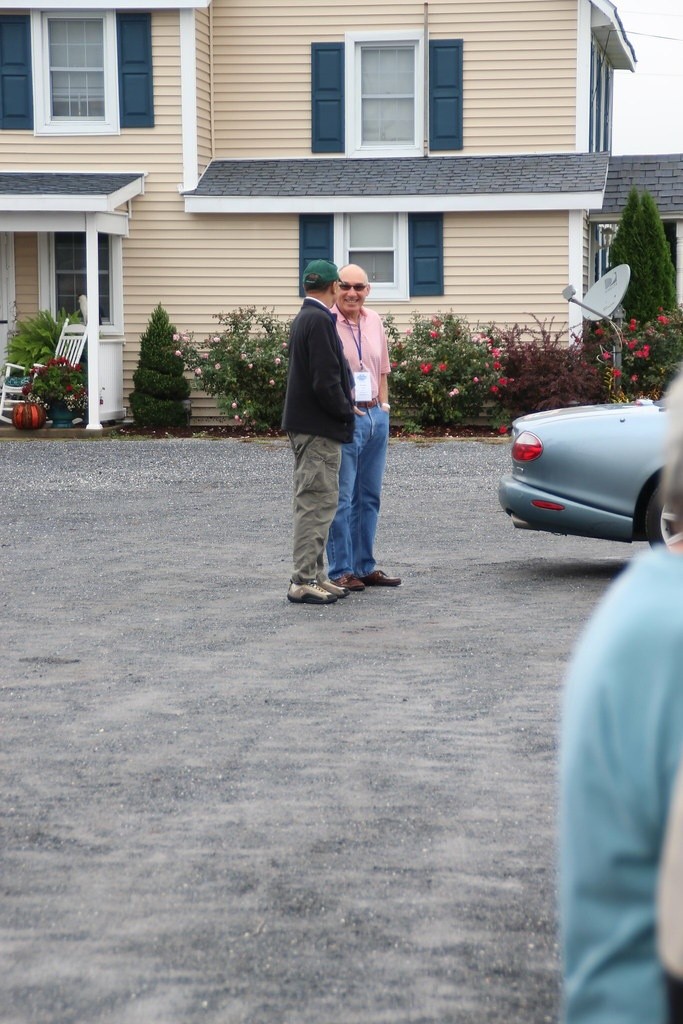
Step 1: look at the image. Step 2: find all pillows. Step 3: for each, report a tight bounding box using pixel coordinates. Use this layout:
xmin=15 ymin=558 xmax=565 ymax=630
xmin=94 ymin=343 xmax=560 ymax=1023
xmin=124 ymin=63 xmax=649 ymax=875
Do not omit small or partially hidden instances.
xmin=5 ymin=376 xmax=30 ymax=400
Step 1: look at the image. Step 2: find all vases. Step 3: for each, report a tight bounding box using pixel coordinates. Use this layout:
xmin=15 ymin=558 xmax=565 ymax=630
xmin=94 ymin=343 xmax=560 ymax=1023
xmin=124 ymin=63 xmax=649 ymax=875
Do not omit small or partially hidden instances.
xmin=46 ymin=401 xmax=82 ymax=428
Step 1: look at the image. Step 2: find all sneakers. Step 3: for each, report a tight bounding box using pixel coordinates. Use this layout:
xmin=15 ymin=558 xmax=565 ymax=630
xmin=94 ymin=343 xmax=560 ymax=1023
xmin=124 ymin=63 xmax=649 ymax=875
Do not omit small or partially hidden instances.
xmin=287 ymin=579 xmax=337 ymax=604
xmin=318 ymin=580 xmax=351 ymax=598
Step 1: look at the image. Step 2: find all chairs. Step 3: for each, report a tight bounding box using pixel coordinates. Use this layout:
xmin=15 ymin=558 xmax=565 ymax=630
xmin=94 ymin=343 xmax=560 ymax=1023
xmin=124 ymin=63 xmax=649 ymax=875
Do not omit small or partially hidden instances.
xmin=0 ymin=318 xmax=88 ymax=428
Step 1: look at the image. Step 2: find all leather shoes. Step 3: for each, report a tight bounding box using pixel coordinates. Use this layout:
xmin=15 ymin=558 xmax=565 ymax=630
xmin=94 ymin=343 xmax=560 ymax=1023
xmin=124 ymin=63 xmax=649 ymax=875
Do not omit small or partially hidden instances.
xmin=330 ymin=574 xmax=366 ymax=591
xmin=358 ymin=569 xmax=401 ymax=586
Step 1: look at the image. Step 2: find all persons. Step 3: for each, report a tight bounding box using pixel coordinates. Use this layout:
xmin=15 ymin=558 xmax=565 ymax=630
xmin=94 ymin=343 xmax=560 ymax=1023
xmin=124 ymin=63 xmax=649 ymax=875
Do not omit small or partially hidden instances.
xmin=326 ymin=264 xmax=401 ymax=590
xmin=556 ymin=361 xmax=683 ymax=1024
xmin=281 ymin=258 xmax=357 ymax=602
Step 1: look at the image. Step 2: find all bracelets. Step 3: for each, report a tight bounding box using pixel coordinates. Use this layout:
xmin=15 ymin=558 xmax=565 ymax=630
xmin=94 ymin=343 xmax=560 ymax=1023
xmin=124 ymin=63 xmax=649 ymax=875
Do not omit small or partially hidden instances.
xmin=379 ymin=403 xmax=391 ymax=410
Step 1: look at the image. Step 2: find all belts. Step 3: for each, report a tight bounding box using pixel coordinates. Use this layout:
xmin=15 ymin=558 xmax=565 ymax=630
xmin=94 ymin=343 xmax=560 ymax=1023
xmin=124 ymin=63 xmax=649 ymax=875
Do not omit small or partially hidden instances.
xmin=354 ymin=396 xmax=380 ymax=409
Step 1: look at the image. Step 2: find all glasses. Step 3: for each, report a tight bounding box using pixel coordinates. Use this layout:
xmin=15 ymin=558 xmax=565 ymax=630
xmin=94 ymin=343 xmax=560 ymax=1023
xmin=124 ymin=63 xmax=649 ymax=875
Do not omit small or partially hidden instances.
xmin=338 ymin=282 xmax=368 ymax=292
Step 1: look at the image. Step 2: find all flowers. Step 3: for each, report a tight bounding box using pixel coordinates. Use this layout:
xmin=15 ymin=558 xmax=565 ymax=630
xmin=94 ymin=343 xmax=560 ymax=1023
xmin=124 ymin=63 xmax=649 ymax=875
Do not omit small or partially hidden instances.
xmin=22 ymin=357 xmax=88 ymax=417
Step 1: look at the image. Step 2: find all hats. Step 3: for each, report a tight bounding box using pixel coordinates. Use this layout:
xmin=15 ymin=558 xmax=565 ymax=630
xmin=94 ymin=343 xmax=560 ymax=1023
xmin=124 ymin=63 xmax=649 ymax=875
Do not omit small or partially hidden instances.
xmin=302 ymin=259 xmax=345 ymax=285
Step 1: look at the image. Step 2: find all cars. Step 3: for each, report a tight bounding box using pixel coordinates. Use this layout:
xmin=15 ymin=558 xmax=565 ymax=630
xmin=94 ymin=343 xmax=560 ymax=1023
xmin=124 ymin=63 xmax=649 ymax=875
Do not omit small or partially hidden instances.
xmin=499 ymin=400 xmax=683 ymax=550
